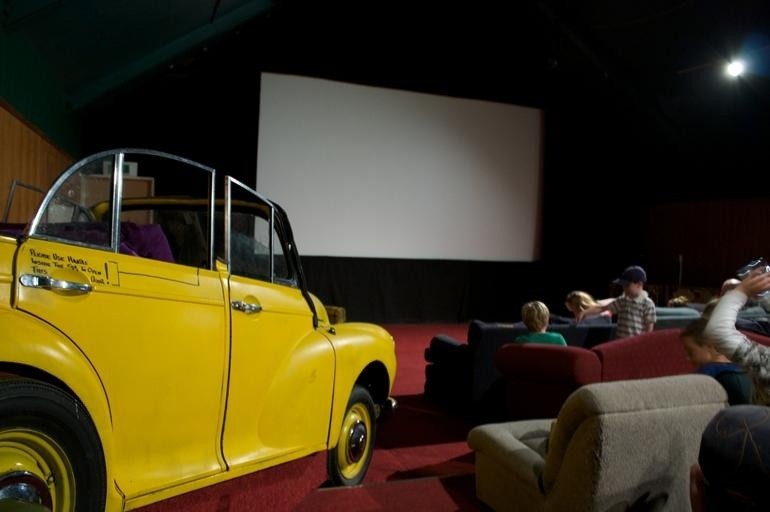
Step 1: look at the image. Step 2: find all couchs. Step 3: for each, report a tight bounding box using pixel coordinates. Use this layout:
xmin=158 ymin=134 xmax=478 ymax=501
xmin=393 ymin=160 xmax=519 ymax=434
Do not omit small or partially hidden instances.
xmin=480 ymin=342 xmax=601 ymax=421
xmin=590 ymin=325 xmax=769 ymax=382
xmin=426 ymin=313 xmax=590 ymax=425
xmin=465 ymin=374 xmax=728 ymax=510
xmin=714 ymin=368 xmax=755 ymax=405
xmin=589 ymin=307 xmax=702 ymax=349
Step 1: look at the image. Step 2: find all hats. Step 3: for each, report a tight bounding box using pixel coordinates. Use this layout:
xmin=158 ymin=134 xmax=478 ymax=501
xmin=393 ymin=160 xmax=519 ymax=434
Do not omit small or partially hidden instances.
xmin=614 ymin=266 xmax=648 ymax=285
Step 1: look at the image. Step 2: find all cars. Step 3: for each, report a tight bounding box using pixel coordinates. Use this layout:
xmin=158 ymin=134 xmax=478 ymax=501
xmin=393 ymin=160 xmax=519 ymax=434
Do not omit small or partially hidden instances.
xmin=2 ymin=149 xmax=398 ymax=512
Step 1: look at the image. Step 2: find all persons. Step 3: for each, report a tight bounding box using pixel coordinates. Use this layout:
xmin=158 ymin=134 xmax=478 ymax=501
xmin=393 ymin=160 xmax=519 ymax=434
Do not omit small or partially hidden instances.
xmin=679 ymin=257 xmax=770 ymax=512
xmin=514 ymin=265 xmax=688 ymax=346
